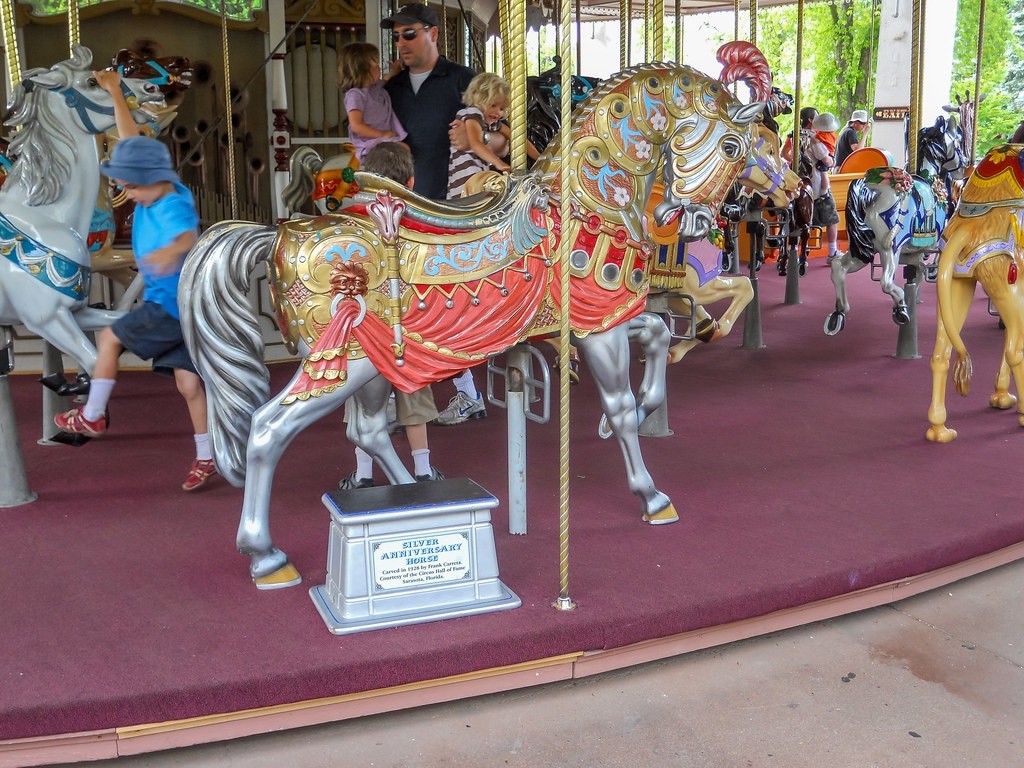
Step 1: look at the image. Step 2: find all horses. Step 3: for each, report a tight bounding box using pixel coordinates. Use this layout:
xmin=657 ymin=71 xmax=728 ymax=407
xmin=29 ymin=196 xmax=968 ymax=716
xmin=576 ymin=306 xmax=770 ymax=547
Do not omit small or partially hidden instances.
xmin=0 ymin=44 xmax=168 ymax=427
xmin=718 ymin=87 xmax=815 ymax=276
xmin=110 ymin=48 xmax=194 ymax=242
xmin=175 ymin=42 xmax=805 ymax=590
xmin=282 ymin=124 xmax=803 ymax=492
xmin=526 ymin=55 xmax=603 ymax=169
xmin=925 ymin=122 xmax=1024 ymax=443
xmin=823 ymin=115 xmax=968 ymax=336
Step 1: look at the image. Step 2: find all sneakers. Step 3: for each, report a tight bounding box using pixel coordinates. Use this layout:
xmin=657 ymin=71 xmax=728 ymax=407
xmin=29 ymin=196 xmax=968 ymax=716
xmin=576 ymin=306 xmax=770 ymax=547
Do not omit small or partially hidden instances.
xmin=386 ymin=396 xmax=405 ymax=433
xmin=182 ymin=457 xmax=218 ymax=491
xmin=432 ymin=391 xmax=488 ymax=425
xmin=54 ymin=408 xmax=107 ymax=437
xmin=337 ymin=470 xmax=374 ymax=490
xmin=415 ymin=466 xmax=445 ymax=481
xmin=826 ymin=249 xmax=845 ymax=264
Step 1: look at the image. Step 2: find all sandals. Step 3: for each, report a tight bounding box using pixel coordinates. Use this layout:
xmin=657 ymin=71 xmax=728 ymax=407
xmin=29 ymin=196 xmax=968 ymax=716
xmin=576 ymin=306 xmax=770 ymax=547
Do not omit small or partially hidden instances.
xmin=816 ymin=193 xmax=830 ymax=204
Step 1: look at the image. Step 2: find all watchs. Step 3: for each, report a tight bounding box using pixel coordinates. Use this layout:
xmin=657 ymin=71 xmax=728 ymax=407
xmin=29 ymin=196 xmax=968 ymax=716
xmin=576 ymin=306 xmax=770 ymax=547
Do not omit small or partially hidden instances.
xmin=483 ymin=131 xmax=491 ymax=144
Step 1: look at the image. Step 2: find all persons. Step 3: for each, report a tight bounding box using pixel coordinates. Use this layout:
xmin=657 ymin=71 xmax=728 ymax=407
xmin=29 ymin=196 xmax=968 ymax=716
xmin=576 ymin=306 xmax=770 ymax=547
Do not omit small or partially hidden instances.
xmin=781 ymin=107 xmax=845 ymax=263
xmin=53 ymin=70 xmax=216 ymax=491
xmin=832 ymin=110 xmax=871 ymax=174
xmin=379 ymin=3 xmax=488 ymax=425
xmin=447 ymin=73 xmax=541 ymax=201
xmin=337 ymin=141 xmax=445 ymax=491
xmin=335 ymin=44 xmax=403 ymax=163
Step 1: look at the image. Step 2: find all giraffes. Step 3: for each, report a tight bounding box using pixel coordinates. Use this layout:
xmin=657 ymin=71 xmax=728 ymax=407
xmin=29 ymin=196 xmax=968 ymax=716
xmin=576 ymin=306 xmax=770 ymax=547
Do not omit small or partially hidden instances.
xmin=942 ymin=90 xmax=987 ymax=167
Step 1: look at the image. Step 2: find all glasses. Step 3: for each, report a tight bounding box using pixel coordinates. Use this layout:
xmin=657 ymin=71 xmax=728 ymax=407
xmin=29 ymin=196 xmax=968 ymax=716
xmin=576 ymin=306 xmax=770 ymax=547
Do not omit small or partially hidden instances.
xmin=857 ymin=121 xmax=867 ymax=125
xmin=392 ymin=26 xmax=428 ymax=42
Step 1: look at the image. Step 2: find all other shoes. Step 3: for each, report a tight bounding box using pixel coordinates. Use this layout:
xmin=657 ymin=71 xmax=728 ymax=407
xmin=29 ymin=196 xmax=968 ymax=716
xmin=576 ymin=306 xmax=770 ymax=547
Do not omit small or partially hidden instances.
xmin=769 ymin=249 xmax=779 ymax=261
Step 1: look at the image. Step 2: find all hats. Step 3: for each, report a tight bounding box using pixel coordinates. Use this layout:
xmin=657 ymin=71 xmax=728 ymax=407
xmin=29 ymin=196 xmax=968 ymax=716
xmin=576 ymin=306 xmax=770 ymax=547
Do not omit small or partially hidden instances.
xmin=101 ymin=136 xmax=181 ymax=185
xmin=848 ymin=110 xmax=868 ymax=123
xmin=380 ymin=3 xmax=440 ymax=29
xmin=812 ymin=112 xmax=840 ymax=132
